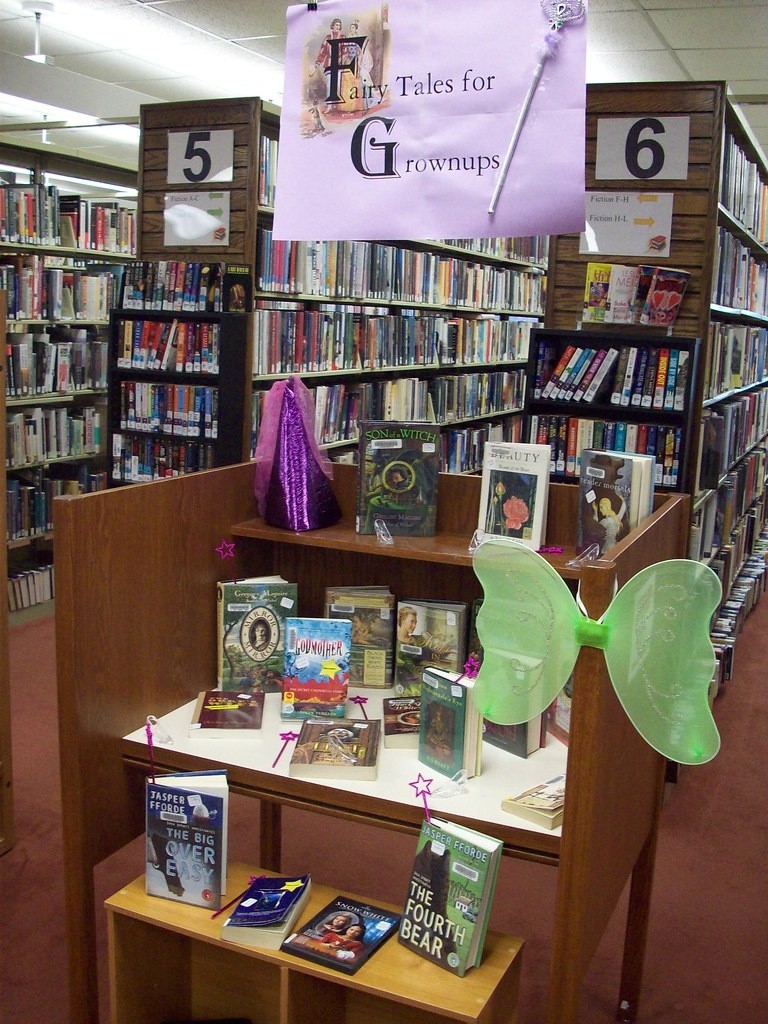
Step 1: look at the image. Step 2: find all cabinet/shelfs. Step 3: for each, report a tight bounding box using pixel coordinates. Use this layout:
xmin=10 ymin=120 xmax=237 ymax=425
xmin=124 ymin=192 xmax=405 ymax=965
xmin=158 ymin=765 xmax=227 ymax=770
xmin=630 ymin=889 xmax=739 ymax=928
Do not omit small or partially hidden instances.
xmin=519 ymin=329 xmax=700 ymax=509
xmin=51 ymin=461 xmax=690 ymax=1024
xmin=0 ymin=239 xmax=136 ymax=548
xmin=104 ymin=308 xmax=247 ymax=490
xmin=543 ymin=81 xmax=768 ymax=784
xmin=136 ymin=96 xmax=548 ymax=475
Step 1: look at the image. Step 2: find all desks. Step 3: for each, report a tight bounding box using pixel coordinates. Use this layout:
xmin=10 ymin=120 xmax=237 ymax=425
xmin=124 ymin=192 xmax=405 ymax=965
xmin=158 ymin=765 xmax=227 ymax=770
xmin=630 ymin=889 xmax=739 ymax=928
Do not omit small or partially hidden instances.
xmin=103 ymin=858 xmax=528 ymax=1024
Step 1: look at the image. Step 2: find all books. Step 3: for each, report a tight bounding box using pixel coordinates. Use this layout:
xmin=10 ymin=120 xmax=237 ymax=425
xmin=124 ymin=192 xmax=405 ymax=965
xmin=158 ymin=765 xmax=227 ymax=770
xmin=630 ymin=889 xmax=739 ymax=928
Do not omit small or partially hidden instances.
xmin=687 ymin=133 xmax=768 ymax=712
xmin=143 ymin=420 xmax=657 ymax=975
xmin=251 ymin=135 xmax=550 ymax=475
xmin=0 ymin=181 xmax=251 ymax=613
xmin=534 ymin=262 xmax=691 ymax=490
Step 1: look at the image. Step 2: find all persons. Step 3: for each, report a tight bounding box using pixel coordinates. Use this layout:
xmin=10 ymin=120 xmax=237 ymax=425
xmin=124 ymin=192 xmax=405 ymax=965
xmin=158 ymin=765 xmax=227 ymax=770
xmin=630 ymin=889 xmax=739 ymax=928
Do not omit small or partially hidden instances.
xmin=321 ymin=921 xmax=366 ymax=964
xmin=317 ymin=914 xmax=351 ymax=939
xmin=396 ymin=605 xmax=420 ymax=643
